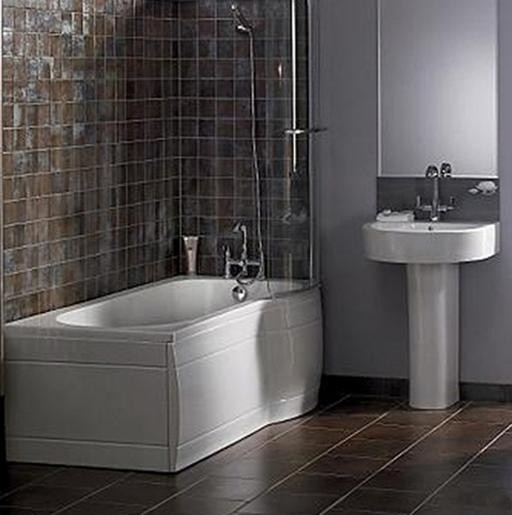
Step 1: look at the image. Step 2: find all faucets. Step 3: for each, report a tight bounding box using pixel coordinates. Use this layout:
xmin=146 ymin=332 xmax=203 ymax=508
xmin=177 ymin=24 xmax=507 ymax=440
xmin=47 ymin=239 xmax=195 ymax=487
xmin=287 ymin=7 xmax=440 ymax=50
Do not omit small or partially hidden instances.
xmin=232 ymin=222 xmax=248 ymax=257
xmin=441 ymin=162 xmax=451 ymax=176
xmin=425 ymin=165 xmax=440 ymax=204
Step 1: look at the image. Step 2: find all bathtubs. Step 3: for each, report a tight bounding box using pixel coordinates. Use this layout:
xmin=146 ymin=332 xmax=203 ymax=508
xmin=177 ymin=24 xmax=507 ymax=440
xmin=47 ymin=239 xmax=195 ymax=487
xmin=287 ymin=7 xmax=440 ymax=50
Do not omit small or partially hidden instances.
xmin=6 ymin=275 xmax=317 ymax=343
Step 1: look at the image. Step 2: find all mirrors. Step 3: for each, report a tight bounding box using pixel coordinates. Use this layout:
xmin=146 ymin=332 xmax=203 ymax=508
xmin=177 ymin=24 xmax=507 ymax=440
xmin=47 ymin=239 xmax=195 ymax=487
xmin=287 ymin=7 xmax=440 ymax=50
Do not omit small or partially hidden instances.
xmin=376 ymin=0 xmax=499 ymax=180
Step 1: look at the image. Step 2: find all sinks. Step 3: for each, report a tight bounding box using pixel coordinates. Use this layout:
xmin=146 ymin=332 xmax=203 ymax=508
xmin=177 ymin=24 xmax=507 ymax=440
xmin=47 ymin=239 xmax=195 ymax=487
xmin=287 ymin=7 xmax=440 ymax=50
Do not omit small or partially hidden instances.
xmin=363 ymin=220 xmax=501 ymax=264
